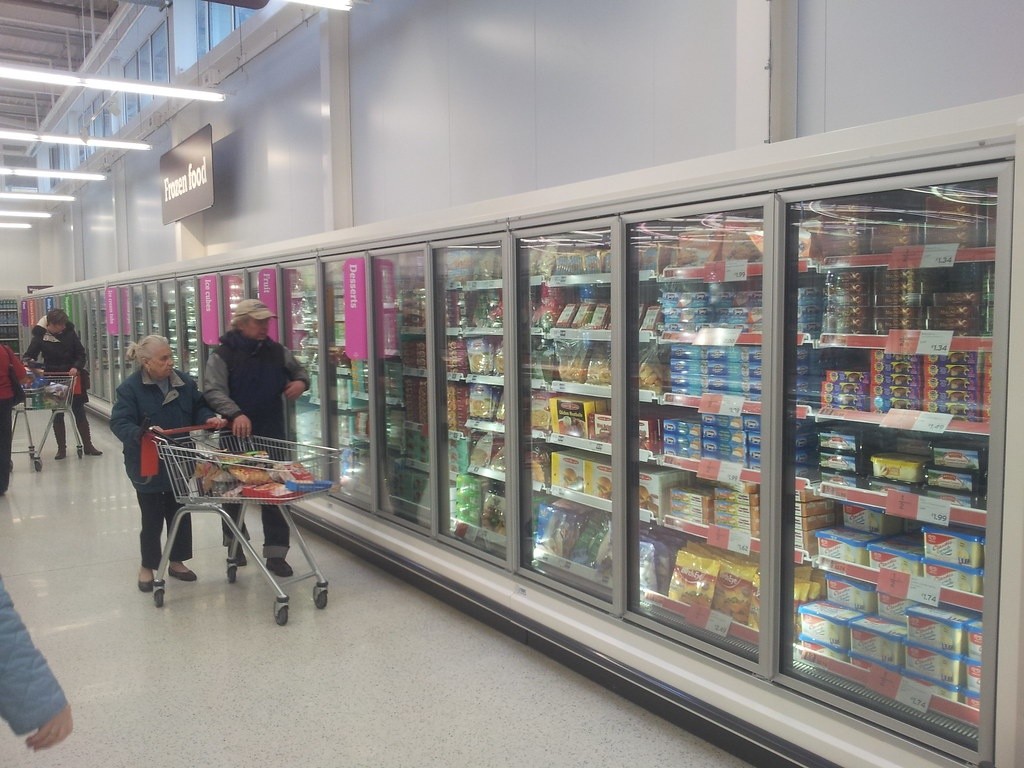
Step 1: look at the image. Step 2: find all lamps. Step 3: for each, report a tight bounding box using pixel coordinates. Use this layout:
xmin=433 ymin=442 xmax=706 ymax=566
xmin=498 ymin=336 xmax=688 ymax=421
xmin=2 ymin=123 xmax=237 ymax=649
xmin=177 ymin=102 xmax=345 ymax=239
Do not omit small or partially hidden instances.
xmin=0 ymin=0 xmax=227 ymax=102
xmin=0 ymin=117 xmax=154 ymax=150
xmin=0 ymin=190 xmax=77 ymax=201
xmin=0 ymin=158 xmax=108 ymax=181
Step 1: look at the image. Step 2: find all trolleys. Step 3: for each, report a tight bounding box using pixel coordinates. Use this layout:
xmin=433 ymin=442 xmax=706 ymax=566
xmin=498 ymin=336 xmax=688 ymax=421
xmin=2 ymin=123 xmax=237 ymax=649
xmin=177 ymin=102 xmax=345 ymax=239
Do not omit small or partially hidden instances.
xmin=146 ymin=424 xmax=346 ymax=626
xmin=8 ymin=371 xmax=85 ymax=473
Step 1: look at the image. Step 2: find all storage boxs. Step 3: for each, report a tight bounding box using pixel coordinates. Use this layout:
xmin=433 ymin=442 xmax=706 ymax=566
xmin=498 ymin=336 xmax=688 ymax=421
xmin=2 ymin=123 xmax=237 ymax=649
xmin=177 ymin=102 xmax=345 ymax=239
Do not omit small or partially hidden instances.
xmin=904 ymin=602 xmax=979 ymax=655
xmin=823 ymin=572 xmax=877 ymax=614
xmin=387 ymin=231 xmax=818 ymax=518
xmin=875 ymin=590 xmax=920 ymax=623
xmin=962 ymin=617 xmax=984 ymax=709
xmin=848 ymin=615 xmax=908 ymax=667
xmin=848 ymin=650 xmax=904 ymax=674
xmin=798 ymin=600 xmax=865 ymax=648
xmin=799 ymin=633 xmax=850 ymax=662
xmin=899 ymin=668 xmax=965 ymax=704
xmin=715 ymin=518 xmax=835 ymax=556
xmin=901 ymin=638 xmax=968 ymax=685
xmin=921 ymin=524 xmax=984 ymax=569
xmin=818 ymin=264 xmax=995 ymax=515
xmin=866 ymin=537 xmax=924 ymax=578
xmin=795 ymin=513 xmax=836 ymax=532
xmin=839 ymin=501 xmax=904 ymax=538
xmin=815 ymin=524 xmax=880 ymax=566
xmin=921 ymin=557 xmax=982 ymax=595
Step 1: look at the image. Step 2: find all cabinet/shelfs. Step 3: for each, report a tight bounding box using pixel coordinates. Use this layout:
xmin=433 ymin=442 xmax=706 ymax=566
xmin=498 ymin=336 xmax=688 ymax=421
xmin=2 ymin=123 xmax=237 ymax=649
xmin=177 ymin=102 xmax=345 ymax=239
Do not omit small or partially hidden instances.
xmin=340 ymin=303 xmax=401 ymax=497
xmin=795 ymin=245 xmax=996 ymax=728
xmin=453 ymin=278 xmax=550 ymax=549
xmin=644 ymin=262 xmax=762 ymax=645
xmin=532 ymin=267 xmax=660 ymax=590
xmin=308 ymin=290 xmax=367 ymax=447
xmin=291 ymin=291 xmax=311 ymax=397
xmin=392 ymin=277 xmax=469 ymax=518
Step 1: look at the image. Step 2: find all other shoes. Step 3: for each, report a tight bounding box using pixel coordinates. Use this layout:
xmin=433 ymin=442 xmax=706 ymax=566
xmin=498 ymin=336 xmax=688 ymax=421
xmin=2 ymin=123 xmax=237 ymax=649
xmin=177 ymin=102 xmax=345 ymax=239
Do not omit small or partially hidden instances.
xmin=266 ymin=558 xmax=293 ymax=577
xmin=168 ymin=566 xmax=197 ymax=581
xmin=138 ymin=579 xmax=154 ymax=593
xmin=228 ymin=547 xmax=247 ymax=566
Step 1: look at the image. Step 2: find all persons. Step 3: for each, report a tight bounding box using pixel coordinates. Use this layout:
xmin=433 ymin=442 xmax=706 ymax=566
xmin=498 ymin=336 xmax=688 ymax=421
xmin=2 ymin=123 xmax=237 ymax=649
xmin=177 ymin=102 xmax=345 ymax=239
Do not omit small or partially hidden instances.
xmin=203 ymin=297 xmax=312 ymax=577
xmin=0 ymin=574 xmax=73 ymax=753
xmin=19 ymin=308 xmax=103 ymax=461
xmin=0 ymin=343 xmax=27 ymax=495
xmin=109 ymin=332 xmax=227 ymax=593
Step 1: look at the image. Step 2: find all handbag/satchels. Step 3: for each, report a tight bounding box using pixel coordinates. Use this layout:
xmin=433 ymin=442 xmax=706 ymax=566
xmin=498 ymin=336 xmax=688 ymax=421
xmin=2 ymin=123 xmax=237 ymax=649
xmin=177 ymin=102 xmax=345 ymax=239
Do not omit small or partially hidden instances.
xmin=3 ymin=345 xmax=25 ymax=406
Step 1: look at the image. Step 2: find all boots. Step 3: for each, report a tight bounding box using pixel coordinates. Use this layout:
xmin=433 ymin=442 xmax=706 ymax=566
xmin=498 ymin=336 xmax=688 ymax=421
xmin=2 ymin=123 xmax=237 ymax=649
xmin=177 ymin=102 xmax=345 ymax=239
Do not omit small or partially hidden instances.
xmin=53 ymin=422 xmax=67 ymax=460
xmin=78 ymin=420 xmax=102 ymax=455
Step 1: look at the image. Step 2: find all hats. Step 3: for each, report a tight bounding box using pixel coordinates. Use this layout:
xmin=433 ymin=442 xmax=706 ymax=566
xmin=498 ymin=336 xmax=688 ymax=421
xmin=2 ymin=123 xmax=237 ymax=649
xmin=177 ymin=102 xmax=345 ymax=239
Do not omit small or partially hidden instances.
xmin=234 ymin=299 xmax=279 ymax=320
